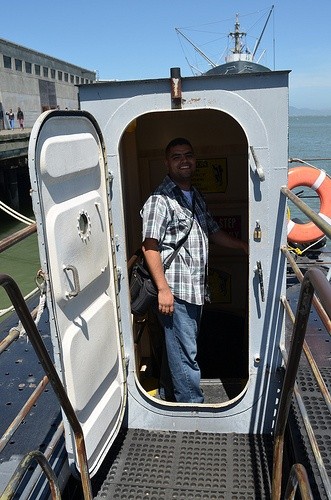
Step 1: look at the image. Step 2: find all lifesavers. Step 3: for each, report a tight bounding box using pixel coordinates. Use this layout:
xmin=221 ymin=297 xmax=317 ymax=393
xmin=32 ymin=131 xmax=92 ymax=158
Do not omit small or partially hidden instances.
xmin=285 ymin=165 xmax=331 ymax=244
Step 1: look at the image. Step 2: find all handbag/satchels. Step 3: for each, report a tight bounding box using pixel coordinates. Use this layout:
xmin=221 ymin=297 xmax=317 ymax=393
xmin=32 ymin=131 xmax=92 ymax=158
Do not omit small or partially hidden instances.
xmin=128 ymin=262 xmax=159 ymax=317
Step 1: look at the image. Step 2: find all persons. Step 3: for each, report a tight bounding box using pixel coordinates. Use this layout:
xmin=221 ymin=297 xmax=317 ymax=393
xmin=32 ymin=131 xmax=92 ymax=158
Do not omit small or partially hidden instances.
xmin=6 ymin=105 xmax=61 ymax=131
xmin=140 ymin=137 xmax=249 ymax=404
